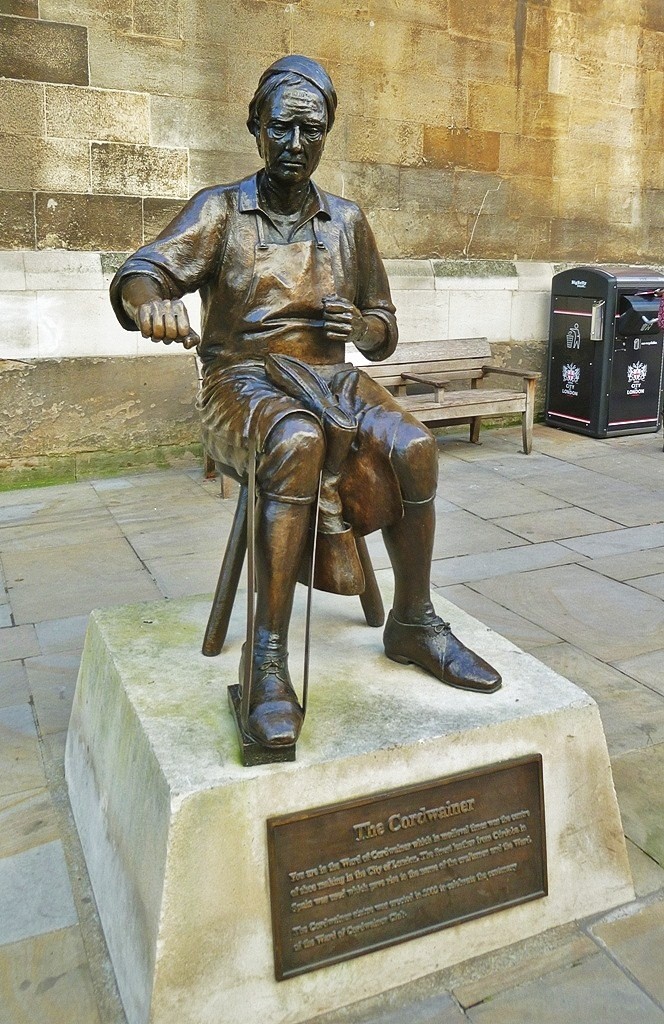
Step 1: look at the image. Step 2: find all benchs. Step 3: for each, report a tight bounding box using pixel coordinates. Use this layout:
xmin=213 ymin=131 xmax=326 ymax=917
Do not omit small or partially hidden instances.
xmin=192 ymin=337 xmax=542 ymax=501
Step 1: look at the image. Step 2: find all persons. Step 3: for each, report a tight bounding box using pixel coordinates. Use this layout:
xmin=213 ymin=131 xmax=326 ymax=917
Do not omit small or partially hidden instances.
xmin=109 ymin=54 xmax=504 ymax=746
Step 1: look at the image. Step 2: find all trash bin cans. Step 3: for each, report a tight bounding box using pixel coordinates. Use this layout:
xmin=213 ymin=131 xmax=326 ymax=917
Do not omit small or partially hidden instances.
xmin=543 ymin=266 xmax=664 ymax=438
